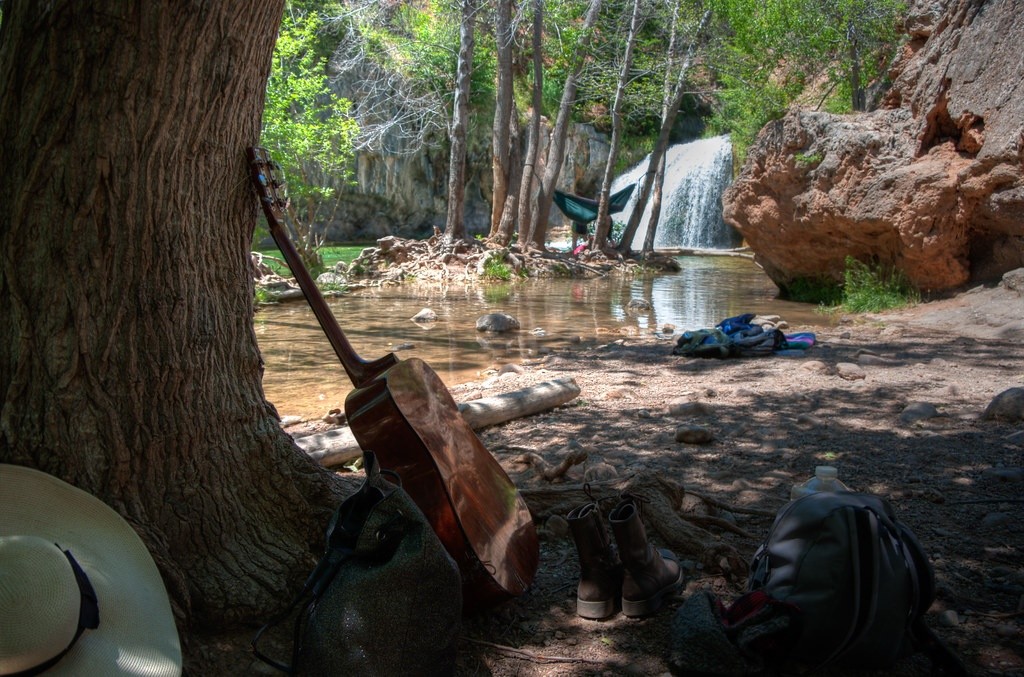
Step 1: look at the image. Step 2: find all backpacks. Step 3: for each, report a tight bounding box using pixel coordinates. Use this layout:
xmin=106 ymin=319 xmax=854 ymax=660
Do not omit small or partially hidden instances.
xmin=721 ymin=491 xmax=974 ymax=677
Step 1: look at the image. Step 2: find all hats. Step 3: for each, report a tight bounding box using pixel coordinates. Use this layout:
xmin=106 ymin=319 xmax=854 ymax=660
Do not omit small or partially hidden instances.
xmin=0 ymin=463 xmax=183 ymax=677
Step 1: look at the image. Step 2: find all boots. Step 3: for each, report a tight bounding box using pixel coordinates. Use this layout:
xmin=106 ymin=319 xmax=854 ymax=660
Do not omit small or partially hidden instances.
xmin=608 ymin=489 xmax=685 ymax=617
xmin=566 ymin=482 xmax=621 ymax=620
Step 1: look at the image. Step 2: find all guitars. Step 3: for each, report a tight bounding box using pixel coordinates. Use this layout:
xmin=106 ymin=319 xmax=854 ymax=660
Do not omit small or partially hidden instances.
xmin=241 ymin=142 xmax=544 ymax=602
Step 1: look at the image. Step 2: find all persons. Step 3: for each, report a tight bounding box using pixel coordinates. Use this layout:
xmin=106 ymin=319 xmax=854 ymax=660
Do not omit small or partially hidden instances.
xmin=571 ymin=212 xmax=617 ymax=252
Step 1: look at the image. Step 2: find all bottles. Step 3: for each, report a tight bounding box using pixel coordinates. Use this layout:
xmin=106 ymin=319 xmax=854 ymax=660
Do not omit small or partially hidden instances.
xmin=800 ymin=465 xmax=848 ymax=493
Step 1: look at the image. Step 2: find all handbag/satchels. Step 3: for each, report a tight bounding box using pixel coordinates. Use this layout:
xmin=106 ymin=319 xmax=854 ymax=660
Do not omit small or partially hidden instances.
xmin=249 ymin=446 xmax=466 ymax=677
